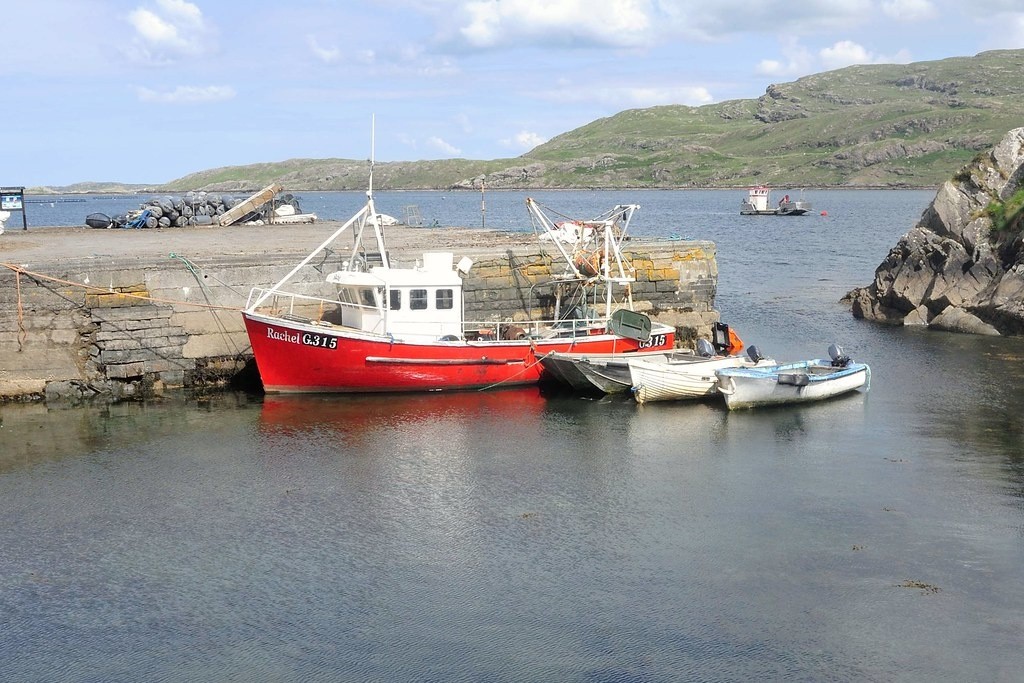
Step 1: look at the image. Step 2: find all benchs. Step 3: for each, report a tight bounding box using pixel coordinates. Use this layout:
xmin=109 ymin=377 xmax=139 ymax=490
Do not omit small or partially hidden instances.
xmin=674 ymin=353 xmax=709 ymax=360
xmin=809 ymin=365 xmax=847 ymax=371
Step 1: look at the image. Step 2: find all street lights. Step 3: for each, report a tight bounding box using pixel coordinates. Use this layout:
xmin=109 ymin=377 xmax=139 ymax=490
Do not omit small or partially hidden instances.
xmin=481 ymin=175 xmax=487 ymax=227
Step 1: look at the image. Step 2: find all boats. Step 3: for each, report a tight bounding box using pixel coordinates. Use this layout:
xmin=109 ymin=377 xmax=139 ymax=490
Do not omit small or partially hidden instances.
xmin=238 ymin=112 xmax=677 ymax=395
xmin=627 ymin=346 xmax=777 ymax=404
xmin=718 ymin=345 xmax=867 ymax=410
xmin=740 ymin=180 xmax=813 ymax=216
xmin=534 ymin=322 xmax=745 ymax=395
